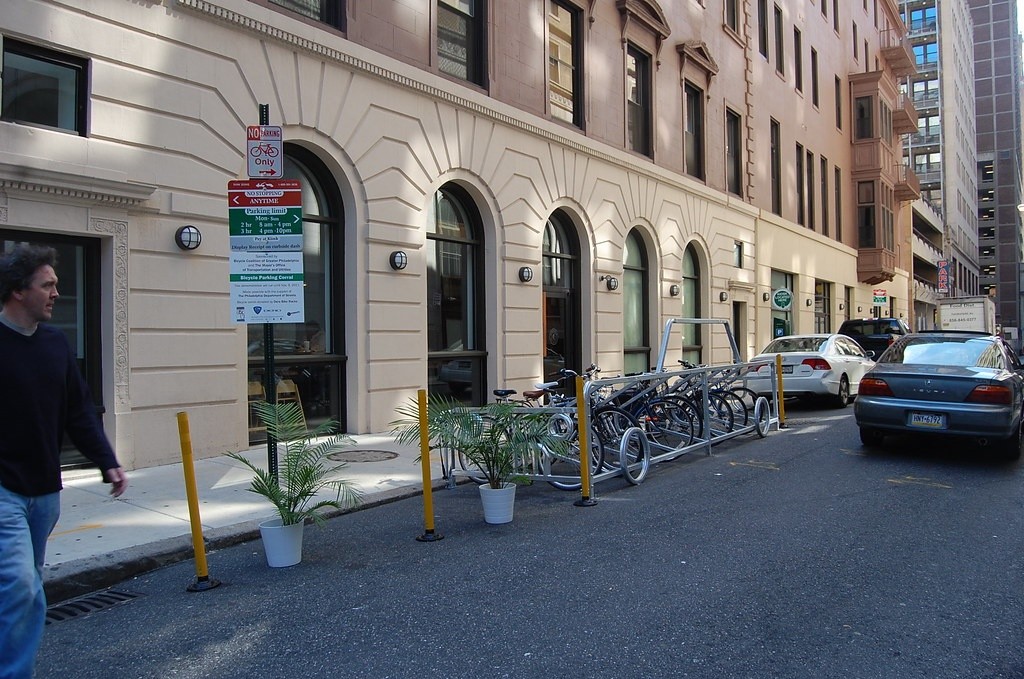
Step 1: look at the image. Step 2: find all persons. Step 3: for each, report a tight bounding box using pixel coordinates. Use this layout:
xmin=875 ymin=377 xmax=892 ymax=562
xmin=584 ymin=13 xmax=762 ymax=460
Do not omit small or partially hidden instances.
xmin=287 ymin=320 xmax=325 ymax=411
xmin=0 ymin=242 xmax=127 ymax=678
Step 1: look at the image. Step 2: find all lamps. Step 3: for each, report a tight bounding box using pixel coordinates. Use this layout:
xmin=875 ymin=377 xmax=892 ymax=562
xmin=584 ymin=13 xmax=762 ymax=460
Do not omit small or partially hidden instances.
xmin=839 ymin=303 xmax=904 ymax=318
xmin=389 ymin=250 xmax=408 ymax=270
xmin=175 ymin=225 xmax=202 ymax=251
xmin=607 ymin=277 xmax=618 ymax=291
xmin=763 ymin=292 xmax=770 ymax=302
xmin=806 ymin=298 xmax=813 ymax=307
xmin=519 ymin=266 xmax=533 ymax=283
xmin=670 ymin=285 xmax=681 ymax=297
xmin=719 ymin=292 xmax=728 ymax=302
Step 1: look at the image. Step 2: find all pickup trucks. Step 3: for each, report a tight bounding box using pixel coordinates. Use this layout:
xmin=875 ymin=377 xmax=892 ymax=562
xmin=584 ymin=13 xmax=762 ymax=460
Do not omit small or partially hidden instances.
xmin=836 ymin=318 xmax=912 ymax=362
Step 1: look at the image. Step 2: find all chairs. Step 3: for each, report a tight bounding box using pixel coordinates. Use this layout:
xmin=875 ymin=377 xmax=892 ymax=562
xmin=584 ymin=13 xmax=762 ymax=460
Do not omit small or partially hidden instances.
xmin=247 ymin=378 xmax=309 ymax=435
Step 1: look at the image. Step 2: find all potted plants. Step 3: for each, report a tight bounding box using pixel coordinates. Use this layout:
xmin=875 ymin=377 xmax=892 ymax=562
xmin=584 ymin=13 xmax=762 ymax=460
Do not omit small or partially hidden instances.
xmin=220 ymin=397 xmax=368 ymax=568
xmin=387 ymin=389 xmax=576 ymax=524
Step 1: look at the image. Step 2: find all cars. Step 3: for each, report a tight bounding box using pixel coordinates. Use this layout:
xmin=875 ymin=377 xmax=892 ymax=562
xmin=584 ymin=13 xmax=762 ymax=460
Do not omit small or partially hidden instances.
xmin=747 ymin=333 xmax=879 ymax=408
xmin=854 ymin=329 xmax=1024 ymax=461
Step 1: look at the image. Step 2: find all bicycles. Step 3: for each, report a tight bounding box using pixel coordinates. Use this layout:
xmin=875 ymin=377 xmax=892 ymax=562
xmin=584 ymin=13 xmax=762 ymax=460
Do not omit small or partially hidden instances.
xmin=459 ymin=356 xmax=770 ymax=495
